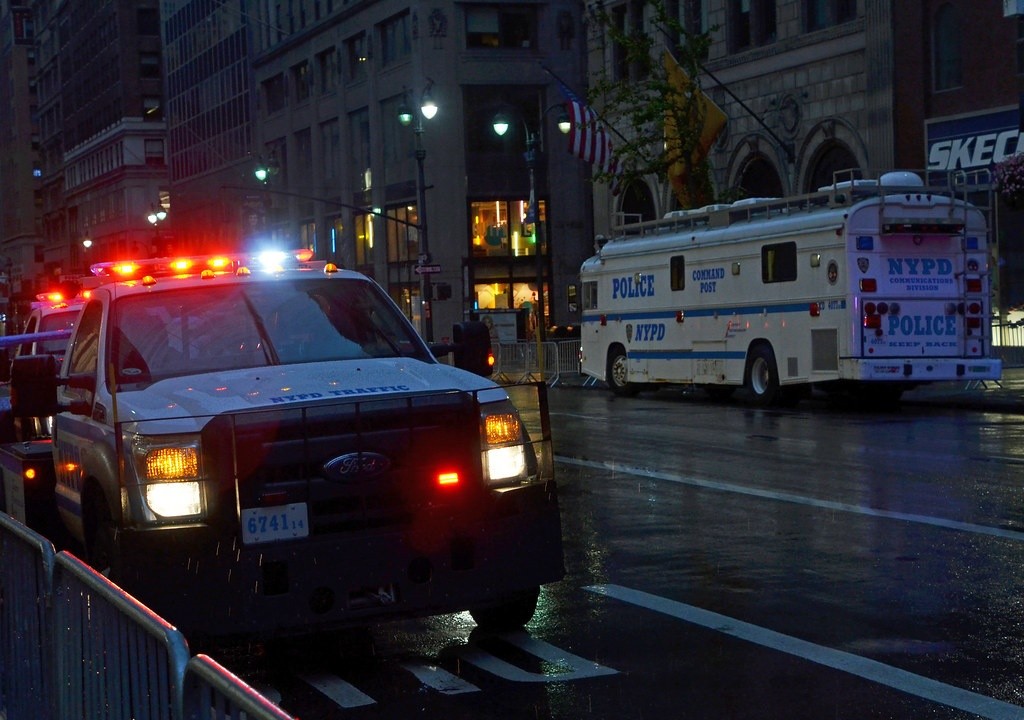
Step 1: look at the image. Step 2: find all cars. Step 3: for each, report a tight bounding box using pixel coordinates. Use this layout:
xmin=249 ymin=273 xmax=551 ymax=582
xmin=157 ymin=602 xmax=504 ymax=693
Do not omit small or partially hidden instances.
xmin=9 ymin=292 xmax=84 ymax=383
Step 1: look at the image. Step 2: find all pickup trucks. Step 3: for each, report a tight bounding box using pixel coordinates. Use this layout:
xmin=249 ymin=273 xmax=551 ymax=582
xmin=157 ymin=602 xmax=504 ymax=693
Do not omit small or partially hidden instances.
xmin=0 ymin=244 xmax=566 ymax=633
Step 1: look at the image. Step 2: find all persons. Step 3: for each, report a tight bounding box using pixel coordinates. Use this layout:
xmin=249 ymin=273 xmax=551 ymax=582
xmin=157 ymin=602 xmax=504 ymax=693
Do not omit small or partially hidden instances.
xmin=481 ymin=315 xmax=500 ymax=343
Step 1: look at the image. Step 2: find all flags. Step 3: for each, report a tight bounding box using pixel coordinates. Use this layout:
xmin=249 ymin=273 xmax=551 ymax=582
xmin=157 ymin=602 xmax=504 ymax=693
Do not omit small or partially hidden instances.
xmin=553 ymin=79 xmax=624 ymax=197
xmin=662 ymin=46 xmax=727 ymax=211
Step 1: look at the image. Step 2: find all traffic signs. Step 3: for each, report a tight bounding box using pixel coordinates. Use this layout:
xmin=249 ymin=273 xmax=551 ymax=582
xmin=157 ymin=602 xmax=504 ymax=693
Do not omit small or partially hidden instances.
xmin=414 ymin=265 xmax=442 ymax=274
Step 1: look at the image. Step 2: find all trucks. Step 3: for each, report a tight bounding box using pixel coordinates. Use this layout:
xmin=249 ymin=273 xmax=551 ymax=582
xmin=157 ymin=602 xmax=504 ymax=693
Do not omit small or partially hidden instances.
xmin=578 ymin=166 xmax=1003 ymax=403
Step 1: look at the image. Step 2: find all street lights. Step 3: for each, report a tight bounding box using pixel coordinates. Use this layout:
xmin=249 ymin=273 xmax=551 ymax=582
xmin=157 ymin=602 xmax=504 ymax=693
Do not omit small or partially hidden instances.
xmin=148 ymin=198 xmax=167 ymax=256
xmin=492 ymin=101 xmax=574 ymax=373
xmin=83 ymin=237 xmax=92 ymax=273
xmin=400 ymin=92 xmax=437 ymax=345
xmin=255 ymin=148 xmax=277 ymax=251
